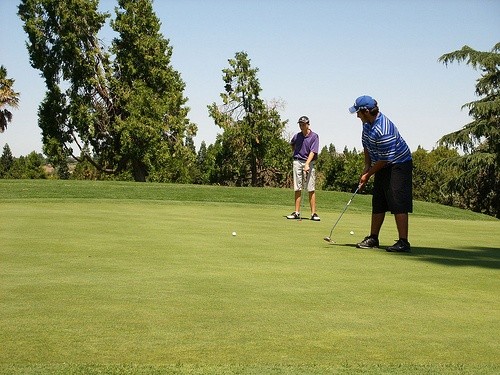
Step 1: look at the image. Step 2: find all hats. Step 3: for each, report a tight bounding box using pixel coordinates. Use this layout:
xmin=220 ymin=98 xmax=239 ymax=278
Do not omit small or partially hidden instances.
xmin=297 ymin=116 xmax=310 ymax=125
xmin=348 ymin=95 xmax=377 ymax=113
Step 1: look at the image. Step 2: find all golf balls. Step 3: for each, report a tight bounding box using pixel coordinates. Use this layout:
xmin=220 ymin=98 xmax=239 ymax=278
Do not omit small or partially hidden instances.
xmin=232 ymin=231 xmax=237 ymax=236
xmin=349 ymin=231 xmax=354 ymax=236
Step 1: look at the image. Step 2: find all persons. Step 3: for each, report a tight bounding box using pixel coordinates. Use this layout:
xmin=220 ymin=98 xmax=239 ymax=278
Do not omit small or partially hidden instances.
xmin=286 ymin=116 xmax=320 ymax=221
xmin=349 ymin=95 xmax=413 ymax=252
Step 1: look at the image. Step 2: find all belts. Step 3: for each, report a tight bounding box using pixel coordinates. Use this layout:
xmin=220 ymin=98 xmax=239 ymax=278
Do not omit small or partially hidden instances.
xmin=294 ymin=157 xmax=307 ymax=161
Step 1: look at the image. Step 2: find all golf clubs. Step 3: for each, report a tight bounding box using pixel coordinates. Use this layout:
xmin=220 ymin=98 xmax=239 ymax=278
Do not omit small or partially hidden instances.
xmin=323 ymin=182 xmax=364 ymax=242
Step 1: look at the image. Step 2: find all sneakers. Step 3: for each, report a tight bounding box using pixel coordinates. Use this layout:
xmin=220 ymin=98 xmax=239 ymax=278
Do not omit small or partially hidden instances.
xmin=386 ymin=239 xmax=411 ymax=253
xmin=356 ymin=236 xmax=379 ymax=249
xmin=286 ymin=211 xmax=300 ymax=219
xmin=311 ymin=212 xmax=320 ymax=221
